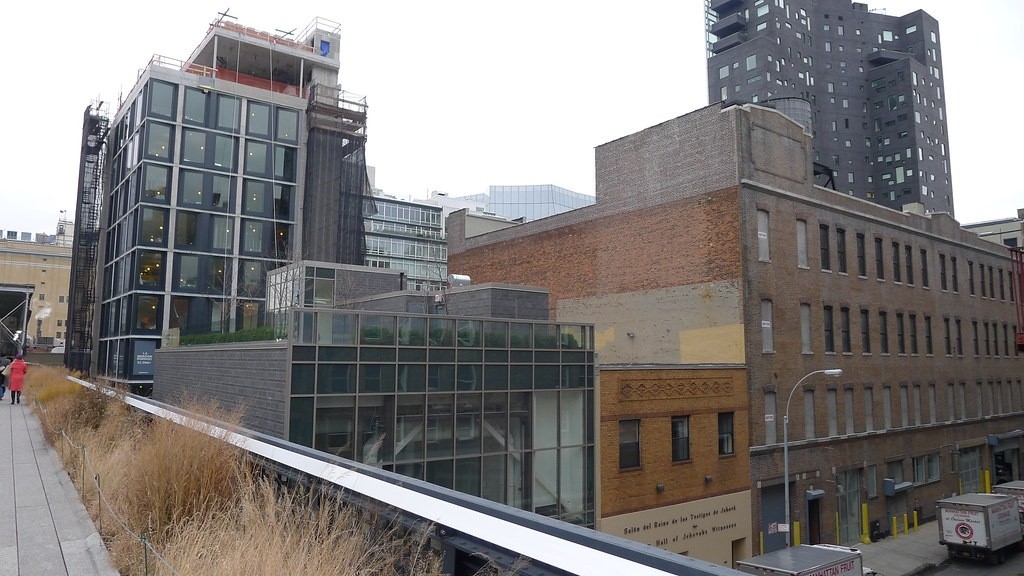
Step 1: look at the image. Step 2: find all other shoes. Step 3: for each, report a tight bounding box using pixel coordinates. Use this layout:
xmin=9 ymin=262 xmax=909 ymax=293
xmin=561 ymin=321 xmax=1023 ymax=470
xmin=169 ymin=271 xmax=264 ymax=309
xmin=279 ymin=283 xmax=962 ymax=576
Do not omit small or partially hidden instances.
xmin=17 ymin=398 xmax=19 ymax=403
xmin=11 ymin=402 xmax=14 ymax=405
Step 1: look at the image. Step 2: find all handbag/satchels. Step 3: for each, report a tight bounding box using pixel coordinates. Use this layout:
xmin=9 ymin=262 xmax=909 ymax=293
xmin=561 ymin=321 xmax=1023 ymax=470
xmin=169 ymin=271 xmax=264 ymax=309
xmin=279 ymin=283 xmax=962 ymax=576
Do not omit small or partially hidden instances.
xmin=2 ymin=362 xmax=13 ymax=378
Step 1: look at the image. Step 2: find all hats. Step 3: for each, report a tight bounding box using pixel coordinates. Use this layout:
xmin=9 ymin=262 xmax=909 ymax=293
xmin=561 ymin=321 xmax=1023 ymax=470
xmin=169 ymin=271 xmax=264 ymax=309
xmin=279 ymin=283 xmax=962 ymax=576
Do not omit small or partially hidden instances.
xmin=16 ymin=354 xmax=22 ymax=360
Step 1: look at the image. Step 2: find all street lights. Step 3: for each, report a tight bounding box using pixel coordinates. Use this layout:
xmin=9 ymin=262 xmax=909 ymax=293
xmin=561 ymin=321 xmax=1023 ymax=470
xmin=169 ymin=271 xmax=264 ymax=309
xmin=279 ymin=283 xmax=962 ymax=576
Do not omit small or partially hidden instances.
xmin=784 ymin=369 xmax=843 ymax=548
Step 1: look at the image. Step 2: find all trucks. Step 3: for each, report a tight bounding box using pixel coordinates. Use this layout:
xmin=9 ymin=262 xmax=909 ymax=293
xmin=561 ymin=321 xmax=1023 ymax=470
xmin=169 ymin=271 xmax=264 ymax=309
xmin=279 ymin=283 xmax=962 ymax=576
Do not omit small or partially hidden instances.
xmin=936 ymin=479 xmax=1024 ymax=565
xmin=735 ymin=544 xmax=882 ymax=576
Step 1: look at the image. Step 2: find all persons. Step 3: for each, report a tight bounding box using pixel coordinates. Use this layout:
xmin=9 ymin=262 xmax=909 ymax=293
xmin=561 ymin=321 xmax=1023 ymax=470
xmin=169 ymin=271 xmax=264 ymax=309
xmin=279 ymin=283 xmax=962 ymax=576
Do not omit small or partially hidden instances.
xmin=0 ymin=353 xmax=28 ymax=405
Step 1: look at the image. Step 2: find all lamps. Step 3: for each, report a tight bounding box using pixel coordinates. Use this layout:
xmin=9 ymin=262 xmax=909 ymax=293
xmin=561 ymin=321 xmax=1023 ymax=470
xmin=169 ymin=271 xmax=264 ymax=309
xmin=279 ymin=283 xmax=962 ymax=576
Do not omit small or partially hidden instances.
xmin=705 ymin=475 xmax=712 ymax=483
xmin=656 ymin=485 xmax=664 ymax=491
